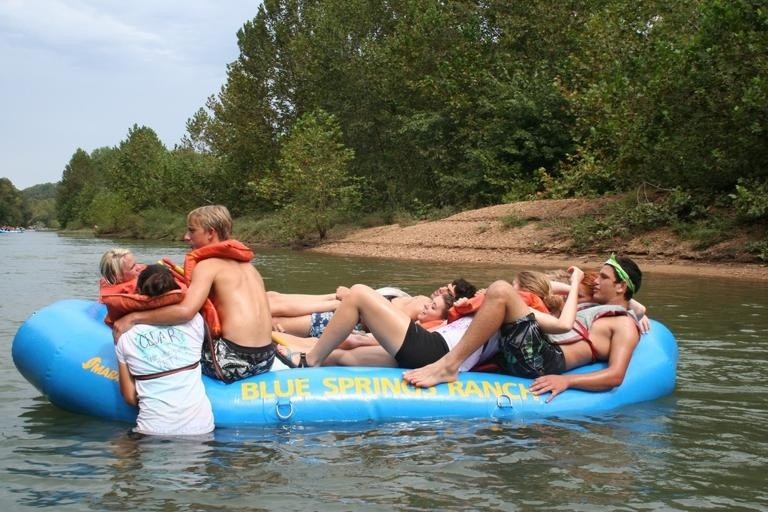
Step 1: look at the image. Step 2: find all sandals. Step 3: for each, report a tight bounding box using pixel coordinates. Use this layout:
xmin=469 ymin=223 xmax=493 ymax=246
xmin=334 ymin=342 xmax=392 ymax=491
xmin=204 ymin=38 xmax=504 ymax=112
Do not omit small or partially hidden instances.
xmin=275 ymin=343 xmax=315 ymax=369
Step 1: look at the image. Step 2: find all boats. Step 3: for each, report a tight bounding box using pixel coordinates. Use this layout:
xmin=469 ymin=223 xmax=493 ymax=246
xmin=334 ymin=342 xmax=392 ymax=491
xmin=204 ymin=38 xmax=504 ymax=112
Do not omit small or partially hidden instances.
xmin=0 ymin=230 xmax=24 ymax=233
xmin=9 ymin=294 xmax=681 ymax=432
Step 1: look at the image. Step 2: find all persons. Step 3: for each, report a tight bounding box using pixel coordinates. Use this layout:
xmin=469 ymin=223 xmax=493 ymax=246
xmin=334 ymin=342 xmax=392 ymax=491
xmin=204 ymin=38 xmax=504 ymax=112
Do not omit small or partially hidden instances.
xmin=0 ymin=223 xmax=23 ymax=234
xmin=265 ymin=251 xmax=653 ymax=404
xmin=98 ymin=248 xmax=141 ymax=287
xmin=114 ymin=263 xmax=217 ymax=437
xmin=113 ymin=203 xmax=274 ymax=383
